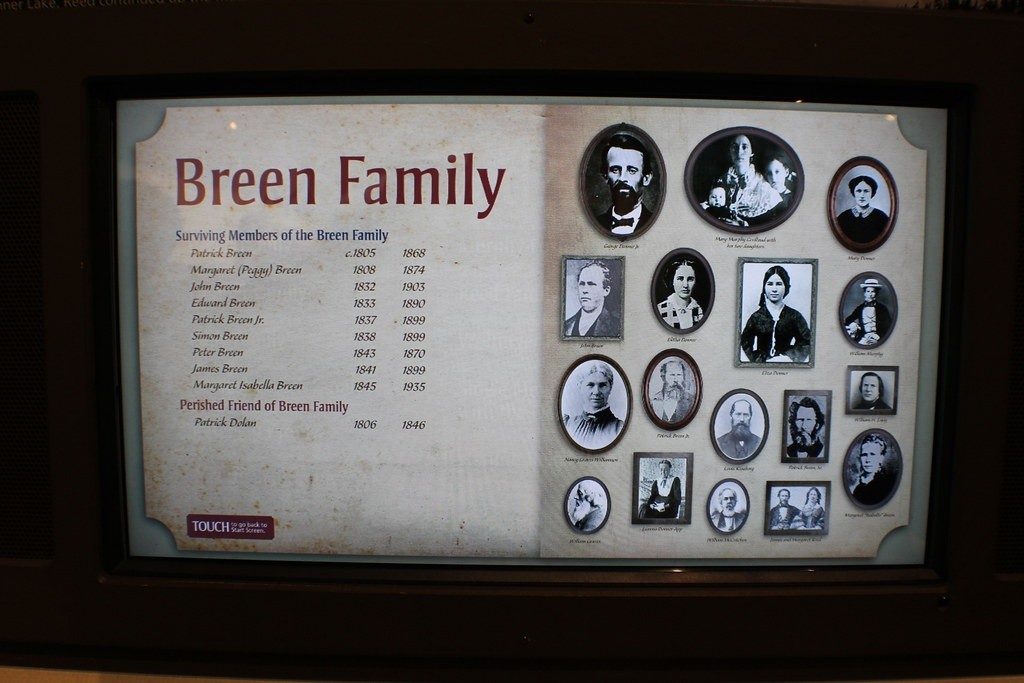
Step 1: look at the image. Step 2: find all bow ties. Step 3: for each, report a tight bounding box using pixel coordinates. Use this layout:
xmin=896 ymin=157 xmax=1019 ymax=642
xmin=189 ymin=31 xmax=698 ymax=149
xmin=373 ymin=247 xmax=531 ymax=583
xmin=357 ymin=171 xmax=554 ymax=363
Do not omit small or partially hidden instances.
xmin=611 ymin=216 xmax=635 ymax=230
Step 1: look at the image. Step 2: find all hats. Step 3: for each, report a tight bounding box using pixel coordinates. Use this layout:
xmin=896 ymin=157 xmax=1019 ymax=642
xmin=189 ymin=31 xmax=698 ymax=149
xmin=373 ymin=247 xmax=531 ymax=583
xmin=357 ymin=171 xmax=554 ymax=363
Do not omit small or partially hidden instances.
xmin=859 ymin=278 xmax=883 ymax=289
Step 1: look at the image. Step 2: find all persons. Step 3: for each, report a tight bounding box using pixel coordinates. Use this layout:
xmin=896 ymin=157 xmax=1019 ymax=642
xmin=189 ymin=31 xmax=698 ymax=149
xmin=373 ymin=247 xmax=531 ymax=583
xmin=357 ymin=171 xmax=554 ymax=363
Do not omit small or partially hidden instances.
xmin=570 ymin=482 xmax=605 ymax=530
xmin=712 ymin=490 xmax=745 ymax=531
xmin=786 ymin=396 xmax=826 ymax=457
xmin=562 ymin=362 xmax=623 ymax=450
xmin=649 ymin=361 xmax=695 ymax=423
xmin=845 ymin=278 xmax=891 ymax=346
xmin=716 ymin=399 xmax=763 ymax=460
xmin=658 ymin=260 xmax=704 ymax=328
xmin=741 ymin=265 xmax=810 ymax=362
xmin=597 ymin=134 xmax=656 ymax=237
xmin=852 ymin=372 xmax=892 ymax=410
xmin=852 ymin=435 xmax=893 ymax=503
xmin=564 ymin=260 xmax=620 ymax=337
xmin=836 ymin=176 xmax=890 ymax=244
xmin=638 ymin=460 xmax=682 ymax=519
xmin=769 ymin=488 xmax=824 ymax=530
xmin=702 ymin=135 xmax=795 ymax=228
